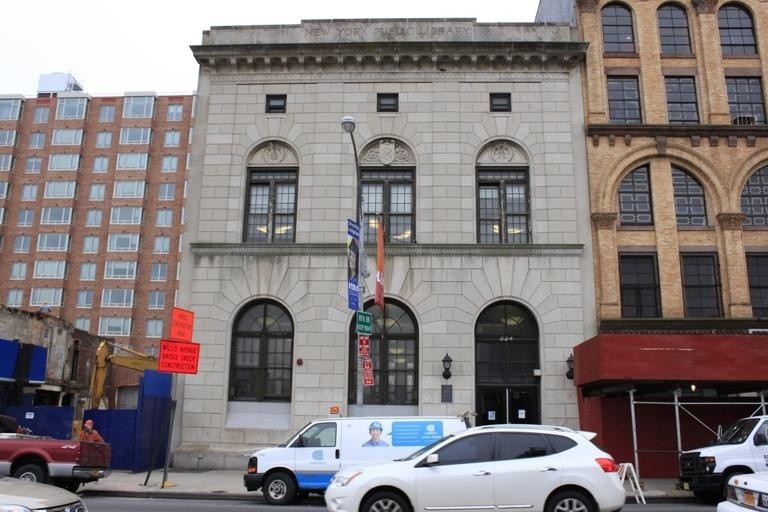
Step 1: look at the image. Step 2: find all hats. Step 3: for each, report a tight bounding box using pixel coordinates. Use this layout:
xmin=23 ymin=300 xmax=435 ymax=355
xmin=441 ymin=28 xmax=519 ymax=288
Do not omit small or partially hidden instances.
xmin=83 ymin=420 xmax=94 ymax=433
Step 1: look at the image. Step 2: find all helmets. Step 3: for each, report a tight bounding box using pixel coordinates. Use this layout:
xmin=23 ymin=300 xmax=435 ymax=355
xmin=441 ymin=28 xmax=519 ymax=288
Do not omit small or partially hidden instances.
xmin=368 ymin=421 xmax=383 ymax=436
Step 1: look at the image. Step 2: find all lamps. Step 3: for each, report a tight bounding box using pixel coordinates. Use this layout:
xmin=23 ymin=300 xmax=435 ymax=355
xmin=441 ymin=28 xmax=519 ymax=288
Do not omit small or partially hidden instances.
xmin=566 ymin=352 xmax=574 ymax=378
xmin=442 ymin=351 xmax=454 ymax=380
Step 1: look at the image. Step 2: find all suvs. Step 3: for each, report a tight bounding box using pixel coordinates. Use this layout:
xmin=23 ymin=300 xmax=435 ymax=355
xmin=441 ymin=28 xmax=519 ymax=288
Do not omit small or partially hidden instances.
xmin=677 ymin=416 xmax=768 ymax=501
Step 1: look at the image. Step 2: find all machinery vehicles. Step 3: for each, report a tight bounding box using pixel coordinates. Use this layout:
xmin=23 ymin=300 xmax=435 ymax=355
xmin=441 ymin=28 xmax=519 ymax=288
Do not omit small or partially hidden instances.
xmin=72 ymin=339 xmax=158 ymax=440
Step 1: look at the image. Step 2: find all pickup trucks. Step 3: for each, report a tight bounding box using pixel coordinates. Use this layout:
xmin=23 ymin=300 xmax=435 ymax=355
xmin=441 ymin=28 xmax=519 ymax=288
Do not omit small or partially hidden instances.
xmin=0 ymin=415 xmax=111 ymax=493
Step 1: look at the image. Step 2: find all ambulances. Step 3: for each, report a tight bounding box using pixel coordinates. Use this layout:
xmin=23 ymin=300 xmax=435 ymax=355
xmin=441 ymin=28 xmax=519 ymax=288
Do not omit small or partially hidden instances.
xmin=243 ymin=405 xmax=464 ymax=505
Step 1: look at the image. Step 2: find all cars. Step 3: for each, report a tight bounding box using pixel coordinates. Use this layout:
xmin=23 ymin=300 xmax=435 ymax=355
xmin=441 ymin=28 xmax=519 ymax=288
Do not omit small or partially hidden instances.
xmin=716 ymin=475 xmax=768 ymax=512
xmin=0 ymin=477 xmax=89 ymax=512
xmin=324 ymin=424 xmax=625 ymax=512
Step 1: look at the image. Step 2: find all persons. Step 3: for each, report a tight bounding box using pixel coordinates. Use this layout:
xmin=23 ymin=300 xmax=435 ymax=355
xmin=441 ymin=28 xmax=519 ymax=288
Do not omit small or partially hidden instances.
xmin=39 ymin=300 xmax=52 ymax=313
xmin=80 ymin=419 xmax=103 ymax=441
xmin=348 ymin=237 xmax=358 ymax=284
xmin=361 ymin=421 xmax=390 ymax=447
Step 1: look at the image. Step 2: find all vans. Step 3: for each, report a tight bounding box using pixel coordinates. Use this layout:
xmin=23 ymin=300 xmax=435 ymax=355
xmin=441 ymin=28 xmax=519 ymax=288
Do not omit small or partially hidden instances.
xmin=341 ymin=117 xmax=363 ymax=404
